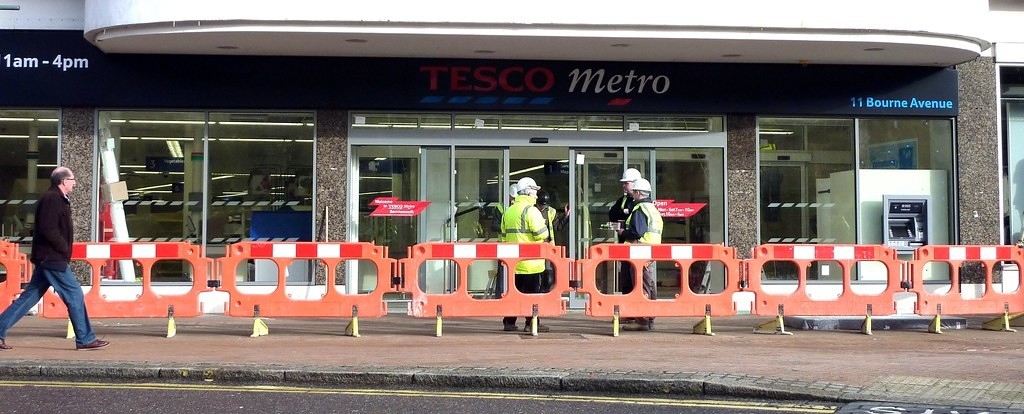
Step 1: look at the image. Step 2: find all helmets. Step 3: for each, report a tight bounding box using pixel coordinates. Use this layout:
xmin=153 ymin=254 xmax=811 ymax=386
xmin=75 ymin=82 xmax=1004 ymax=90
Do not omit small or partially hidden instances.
xmin=538 ymin=191 xmax=550 ymax=204
xmin=509 ymin=184 xmax=519 ymax=198
xmin=630 ymin=178 xmax=652 ymax=191
xmin=620 ymin=168 xmax=641 ymax=181
xmin=517 ymin=177 xmax=541 ymax=193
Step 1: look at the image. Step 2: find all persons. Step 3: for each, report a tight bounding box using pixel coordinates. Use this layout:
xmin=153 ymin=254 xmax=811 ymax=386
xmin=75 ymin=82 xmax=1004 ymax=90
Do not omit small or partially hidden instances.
xmin=491 ymin=177 xmax=571 ymax=332
xmin=0 ymin=167 xmax=109 ymax=351
xmin=608 ymin=168 xmax=641 ymax=324
xmin=617 ymin=179 xmax=664 ymax=331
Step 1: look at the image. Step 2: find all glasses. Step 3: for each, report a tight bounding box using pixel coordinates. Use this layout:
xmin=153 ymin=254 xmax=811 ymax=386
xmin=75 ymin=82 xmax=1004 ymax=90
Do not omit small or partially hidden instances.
xmin=62 ymin=178 xmax=76 ymax=182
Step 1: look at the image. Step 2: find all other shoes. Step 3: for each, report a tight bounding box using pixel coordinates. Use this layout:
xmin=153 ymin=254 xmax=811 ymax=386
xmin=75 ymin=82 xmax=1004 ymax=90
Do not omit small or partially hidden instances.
xmin=623 ymin=320 xmax=655 ymax=330
xmin=0 ymin=338 xmax=12 ymax=349
xmin=503 ymin=322 xmax=519 ymax=331
xmin=612 ymin=318 xmax=635 ymax=323
xmin=524 ymin=324 xmax=549 ymax=332
xmin=77 ymin=336 xmax=109 ymax=350
